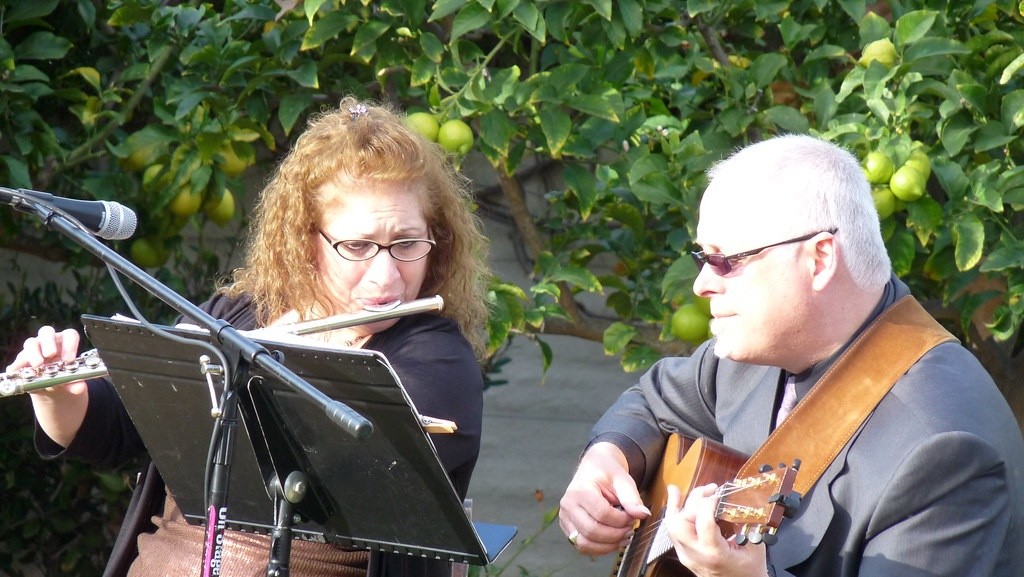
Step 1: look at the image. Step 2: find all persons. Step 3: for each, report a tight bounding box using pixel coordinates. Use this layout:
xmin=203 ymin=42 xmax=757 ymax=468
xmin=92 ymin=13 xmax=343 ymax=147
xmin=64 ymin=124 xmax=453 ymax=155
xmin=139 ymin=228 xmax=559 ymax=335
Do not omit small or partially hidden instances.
xmin=556 ymin=136 xmax=1024 ymax=577
xmin=4 ymin=95 xmax=492 ymax=576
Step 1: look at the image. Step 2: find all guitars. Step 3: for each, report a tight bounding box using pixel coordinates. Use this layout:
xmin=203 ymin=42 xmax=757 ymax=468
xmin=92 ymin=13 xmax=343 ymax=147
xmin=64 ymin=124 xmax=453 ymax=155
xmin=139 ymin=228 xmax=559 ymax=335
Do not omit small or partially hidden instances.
xmin=610 ymin=431 xmax=804 ymax=577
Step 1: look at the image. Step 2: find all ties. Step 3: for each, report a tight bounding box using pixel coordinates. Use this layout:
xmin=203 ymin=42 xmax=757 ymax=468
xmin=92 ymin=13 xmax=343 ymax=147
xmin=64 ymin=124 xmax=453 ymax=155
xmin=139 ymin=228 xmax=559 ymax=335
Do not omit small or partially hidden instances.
xmin=776 ymin=375 xmax=799 ymax=432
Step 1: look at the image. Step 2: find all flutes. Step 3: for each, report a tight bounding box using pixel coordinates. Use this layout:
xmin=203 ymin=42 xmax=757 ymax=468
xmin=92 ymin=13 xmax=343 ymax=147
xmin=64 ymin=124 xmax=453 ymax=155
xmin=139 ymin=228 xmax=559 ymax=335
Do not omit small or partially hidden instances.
xmin=0 ymin=295 xmax=446 ymax=396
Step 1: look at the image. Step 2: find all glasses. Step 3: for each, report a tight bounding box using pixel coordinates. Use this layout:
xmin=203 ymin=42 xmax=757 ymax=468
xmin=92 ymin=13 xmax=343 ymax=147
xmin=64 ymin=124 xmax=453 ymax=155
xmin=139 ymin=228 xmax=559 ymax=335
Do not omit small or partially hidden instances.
xmin=690 ymin=228 xmax=839 ymax=275
xmin=315 ymin=225 xmax=436 ymax=262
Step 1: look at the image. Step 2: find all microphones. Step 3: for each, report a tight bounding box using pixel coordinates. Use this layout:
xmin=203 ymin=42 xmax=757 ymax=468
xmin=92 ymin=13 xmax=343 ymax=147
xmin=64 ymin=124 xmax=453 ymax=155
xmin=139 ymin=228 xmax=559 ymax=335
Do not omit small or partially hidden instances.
xmin=0 ymin=187 xmax=138 ymax=240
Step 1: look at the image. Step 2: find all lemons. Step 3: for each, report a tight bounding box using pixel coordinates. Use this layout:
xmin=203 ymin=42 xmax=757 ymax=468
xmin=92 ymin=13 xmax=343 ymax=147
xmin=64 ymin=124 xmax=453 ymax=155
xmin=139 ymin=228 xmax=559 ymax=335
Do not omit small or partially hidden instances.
xmin=404 ymin=111 xmax=474 ymax=157
xmin=667 ymin=296 xmax=710 ymax=344
xmin=858 ymin=36 xmax=932 ymax=223
xmin=123 ymin=103 xmax=257 ymax=271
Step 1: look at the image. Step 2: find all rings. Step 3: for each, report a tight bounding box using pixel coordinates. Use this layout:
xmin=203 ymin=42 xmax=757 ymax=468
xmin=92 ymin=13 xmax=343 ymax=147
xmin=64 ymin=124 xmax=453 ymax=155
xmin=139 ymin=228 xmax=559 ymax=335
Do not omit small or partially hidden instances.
xmin=566 ymin=529 xmax=581 ymax=545
